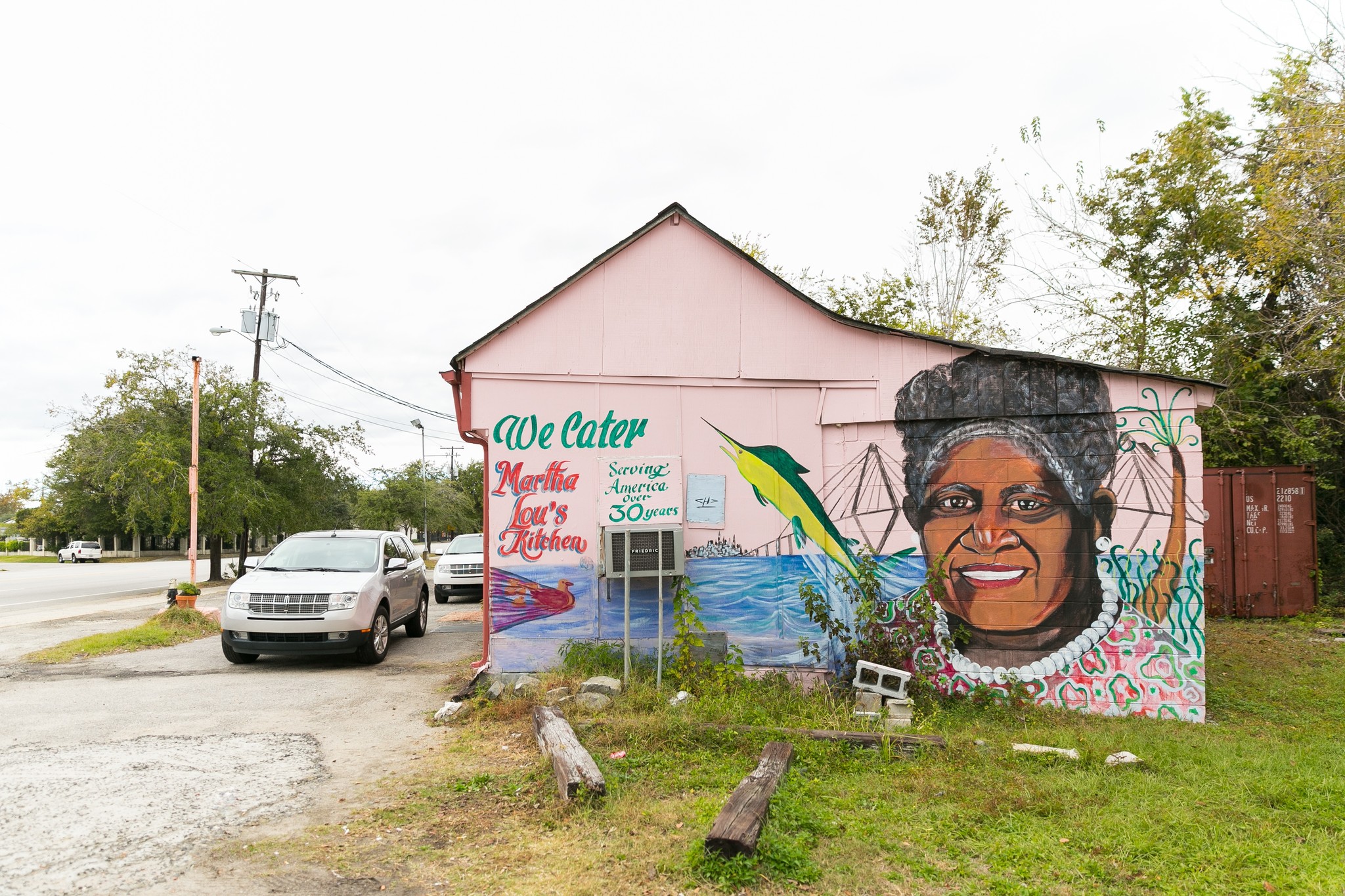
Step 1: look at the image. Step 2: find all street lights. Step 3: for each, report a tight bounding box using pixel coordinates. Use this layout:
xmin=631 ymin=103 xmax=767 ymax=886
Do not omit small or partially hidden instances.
xmin=209 ymin=268 xmax=300 ymax=578
xmin=410 ymin=418 xmax=430 ymax=561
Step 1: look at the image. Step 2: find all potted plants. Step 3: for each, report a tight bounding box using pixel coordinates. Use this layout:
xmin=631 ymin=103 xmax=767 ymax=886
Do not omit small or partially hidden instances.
xmin=175 ymin=582 xmax=201 ymax=609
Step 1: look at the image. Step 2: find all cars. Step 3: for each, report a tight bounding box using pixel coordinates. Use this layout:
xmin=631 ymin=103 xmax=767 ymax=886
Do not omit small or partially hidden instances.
xmin=433 ymin=533 xmax=483 ymax=604
xmin=220 ymin=518 xmax=429 ymax=665
xmin=58 ymin=541 xmax=103 ymax=564
